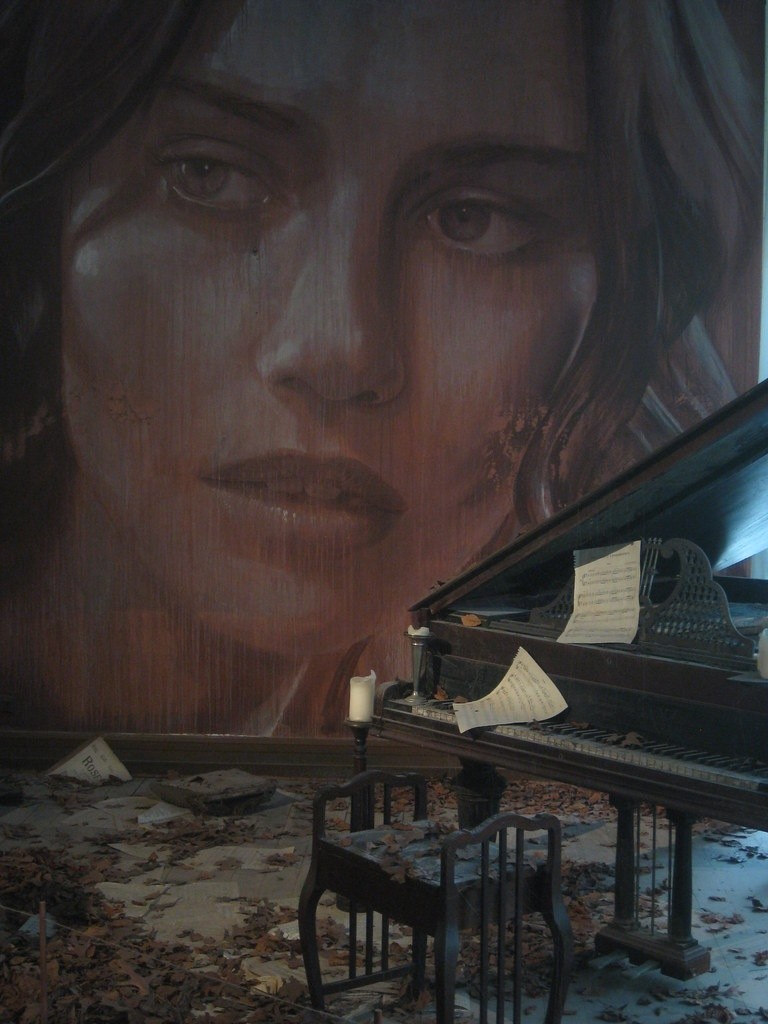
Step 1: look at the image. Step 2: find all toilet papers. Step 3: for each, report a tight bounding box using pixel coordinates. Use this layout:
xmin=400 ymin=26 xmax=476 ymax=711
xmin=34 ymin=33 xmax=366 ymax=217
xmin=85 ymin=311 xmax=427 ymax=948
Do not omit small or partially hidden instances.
xmin=348 ymin=669 xmax=378 ymax=723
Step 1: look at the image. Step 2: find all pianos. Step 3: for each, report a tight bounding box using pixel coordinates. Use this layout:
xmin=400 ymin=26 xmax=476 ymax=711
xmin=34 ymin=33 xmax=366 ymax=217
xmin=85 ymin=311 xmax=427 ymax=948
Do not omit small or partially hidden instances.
xmin=375 ymin=377 xmax=767 ymax=979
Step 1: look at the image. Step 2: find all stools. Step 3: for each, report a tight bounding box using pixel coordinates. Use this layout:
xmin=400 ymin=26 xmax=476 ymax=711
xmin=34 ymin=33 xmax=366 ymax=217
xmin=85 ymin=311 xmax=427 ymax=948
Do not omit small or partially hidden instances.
xmin=298 ymin=771 xmax=574 ymax=1024
